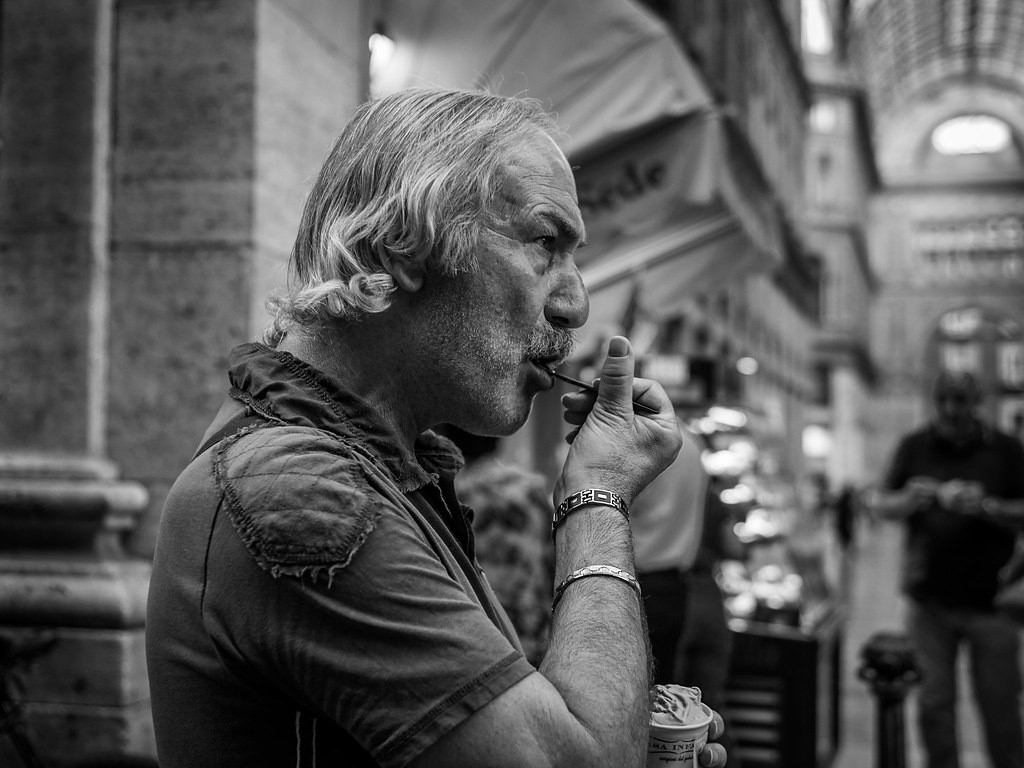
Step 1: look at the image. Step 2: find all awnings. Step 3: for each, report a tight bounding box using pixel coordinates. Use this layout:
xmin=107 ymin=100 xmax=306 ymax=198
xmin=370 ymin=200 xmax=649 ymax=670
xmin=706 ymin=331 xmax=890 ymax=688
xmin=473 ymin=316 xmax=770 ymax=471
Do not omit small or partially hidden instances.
xmin=375 ymin=0 xmax=779 ymax=330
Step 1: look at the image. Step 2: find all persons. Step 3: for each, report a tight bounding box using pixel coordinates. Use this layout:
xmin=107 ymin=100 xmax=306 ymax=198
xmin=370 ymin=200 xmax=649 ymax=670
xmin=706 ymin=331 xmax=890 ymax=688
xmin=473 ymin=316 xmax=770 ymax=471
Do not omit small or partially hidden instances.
xmin=868 ymin=371 xmax=1024 ymax=768
xmin=456 ymin=431 xmax=555 ymax=665
xmin=629 ymin=431 xmax=710 ymax=686
xmin=144 ymin=89 xmax=728 ymax=768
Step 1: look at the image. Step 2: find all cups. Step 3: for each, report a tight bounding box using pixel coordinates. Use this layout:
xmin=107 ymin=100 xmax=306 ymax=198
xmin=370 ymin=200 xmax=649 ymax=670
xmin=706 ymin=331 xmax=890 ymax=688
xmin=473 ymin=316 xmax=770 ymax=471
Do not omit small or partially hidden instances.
xmin=647 ymin=702 xmax=713 ymax=768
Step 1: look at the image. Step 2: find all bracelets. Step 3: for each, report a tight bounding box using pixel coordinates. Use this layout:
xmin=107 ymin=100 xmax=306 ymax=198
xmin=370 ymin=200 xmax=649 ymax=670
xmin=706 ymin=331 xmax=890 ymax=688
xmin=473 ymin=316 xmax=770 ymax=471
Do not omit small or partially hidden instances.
xmin=551 ymin=565 xmax=642 ymax=616
xmin=551 ymin=488 xmax=630 ymax=535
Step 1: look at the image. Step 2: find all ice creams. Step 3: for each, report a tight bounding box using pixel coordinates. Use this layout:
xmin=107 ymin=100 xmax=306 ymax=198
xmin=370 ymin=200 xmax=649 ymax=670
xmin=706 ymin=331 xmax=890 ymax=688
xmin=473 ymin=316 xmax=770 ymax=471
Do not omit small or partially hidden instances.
xmin=643 ymin=684 xmax=713 ymax=768
